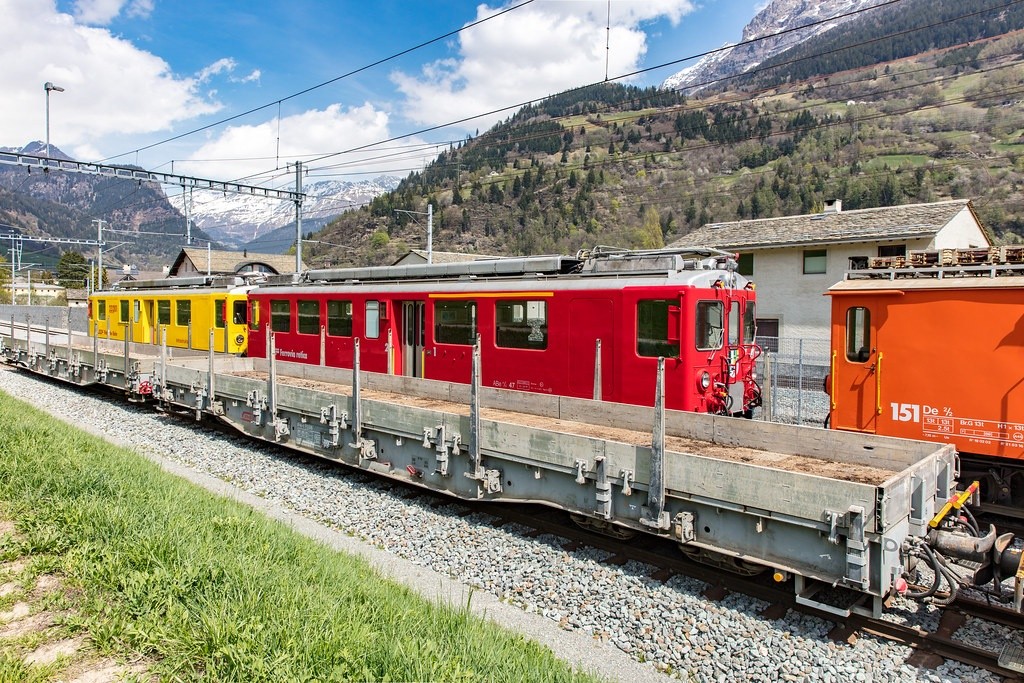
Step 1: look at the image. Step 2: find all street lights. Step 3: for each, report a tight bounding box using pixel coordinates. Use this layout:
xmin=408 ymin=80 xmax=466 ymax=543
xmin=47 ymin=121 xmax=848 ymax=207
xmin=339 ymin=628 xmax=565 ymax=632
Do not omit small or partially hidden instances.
xmin=7 ymin=229 xmax=15 ymax=306
xmin=394 ymin=203 xmax=433 ymax=266
xmin=44 ymin=82 xmax=66 ymax=159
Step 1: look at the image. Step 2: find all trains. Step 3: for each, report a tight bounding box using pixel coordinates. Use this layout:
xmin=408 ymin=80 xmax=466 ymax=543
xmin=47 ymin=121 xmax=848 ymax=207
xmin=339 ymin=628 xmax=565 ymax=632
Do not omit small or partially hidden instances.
xmin=0 ymin=316 xmax=1023 ymax=638
xmin=816 ymin=241 xmax=1024 ymax=533
xmin=86 ymin=242 xmax=764 ymax=426
